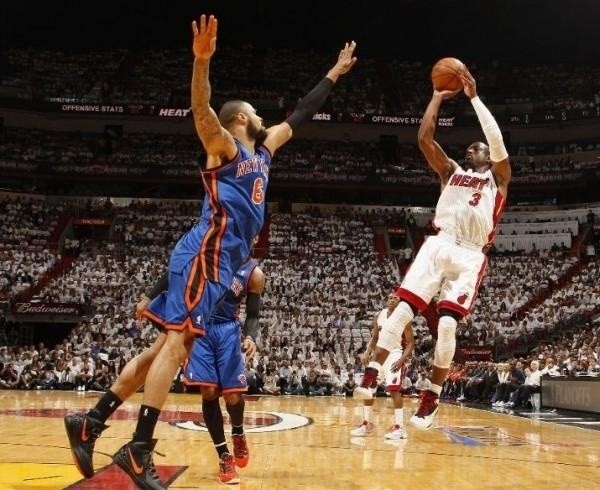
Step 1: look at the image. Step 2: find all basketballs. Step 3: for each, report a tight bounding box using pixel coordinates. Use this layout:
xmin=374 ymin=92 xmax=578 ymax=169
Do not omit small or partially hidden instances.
xmin=432 ymin=57 xmax=464 ymax=90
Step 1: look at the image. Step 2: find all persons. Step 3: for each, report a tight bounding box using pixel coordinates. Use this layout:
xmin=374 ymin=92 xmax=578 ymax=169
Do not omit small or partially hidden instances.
xmin=138 ymin=229 xmax=265 ymax=484
xmin=349 ymin=296 xmax=415 ymax=439
xmin=61 ymin=15 xmax=357 ymax=489
xmin=0 ymin=13 xmax=600 ymax=407
xmin=351 ymin=63 xmax=510 ymax=431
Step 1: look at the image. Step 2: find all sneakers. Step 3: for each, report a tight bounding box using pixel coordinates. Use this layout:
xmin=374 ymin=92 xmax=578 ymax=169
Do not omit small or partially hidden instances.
xmin=410 ymin=389 xmax=439 ymax=431
xmin=64 ymin=413 xmax=110 ymax=478
xmin=350 ymin=420 xmax=375 ymax=436
xmin=492 ymin=400 xmax=514 ymax=407
xmin=384 ymin=424 xmax=407 ymax=440
xmin=353 ymin=367 xmax=386 ymax=399
xmin=113 ymin=438 xmax=169 ymax=490
xmin=218 ymin=433 xmax=249 ymax=484
